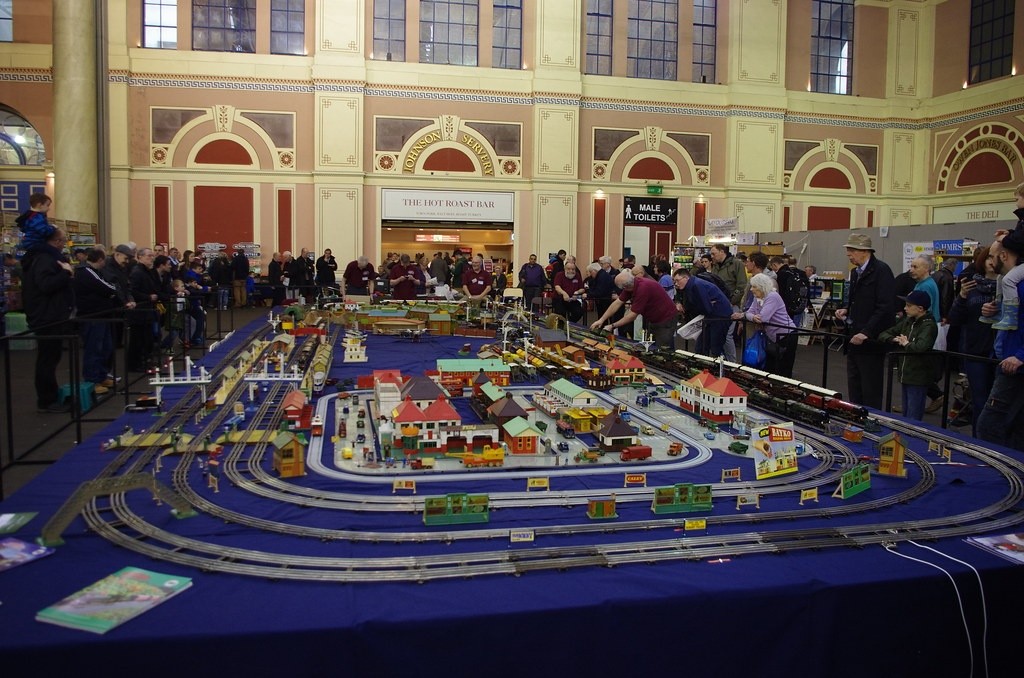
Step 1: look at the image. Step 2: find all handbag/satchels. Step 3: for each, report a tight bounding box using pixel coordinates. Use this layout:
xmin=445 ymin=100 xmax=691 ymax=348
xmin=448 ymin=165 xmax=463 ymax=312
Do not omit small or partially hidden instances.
xmin=742 ymin=322 xmax=768 ymax=370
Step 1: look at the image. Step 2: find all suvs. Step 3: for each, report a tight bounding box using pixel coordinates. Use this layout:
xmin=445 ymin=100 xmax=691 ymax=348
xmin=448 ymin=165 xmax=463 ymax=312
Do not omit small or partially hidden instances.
xmin=587 ymin=447 xmax=606 ymax=456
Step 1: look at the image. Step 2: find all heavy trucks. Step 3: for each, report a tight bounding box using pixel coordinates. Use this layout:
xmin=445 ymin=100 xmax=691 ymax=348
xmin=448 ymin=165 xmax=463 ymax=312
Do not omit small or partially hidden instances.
xmin=297 ymin=324 xmax=325 ymax=372
xmin=613 ymin=401 xmax=631 ymax=422
xmin=619 ymin=446 xmax=653 ymax=461
xmin=233 ymin=400 xmax=246 ymax=421
xmin=409 ymin=457 xmax=434 ymax=470
xmin=222 ymin=415 xmax=243 ymax=434
xmin=555 ymin=419 xmax=575 ymax=439
xmin=727 ymin=440 xmax=747 ymax=454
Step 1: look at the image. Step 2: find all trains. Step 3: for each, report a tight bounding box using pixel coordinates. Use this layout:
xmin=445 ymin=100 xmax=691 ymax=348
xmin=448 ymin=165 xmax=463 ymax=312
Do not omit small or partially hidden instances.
xmin=491 ymin=340 xmax=594 ymax=383
xmin=729 ymin=367 xmax=868 ymax=426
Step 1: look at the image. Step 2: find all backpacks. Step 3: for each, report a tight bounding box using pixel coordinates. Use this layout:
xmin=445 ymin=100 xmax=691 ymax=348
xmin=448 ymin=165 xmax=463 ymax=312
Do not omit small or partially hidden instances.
xmin=774 ymin=264 xmax=809 ymax=316
xmin=545 ymin=261 xmax=558 ymax=278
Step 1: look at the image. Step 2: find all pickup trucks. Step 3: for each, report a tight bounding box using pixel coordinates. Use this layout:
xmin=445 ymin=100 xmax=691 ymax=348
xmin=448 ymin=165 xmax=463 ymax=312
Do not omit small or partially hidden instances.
xmin=556 ymin=440 xmax=569 ymax=452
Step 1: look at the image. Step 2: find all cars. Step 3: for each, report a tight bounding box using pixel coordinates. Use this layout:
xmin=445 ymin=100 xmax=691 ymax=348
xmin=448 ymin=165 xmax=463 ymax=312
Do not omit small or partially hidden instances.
xmin=336 ymin=389 xmax=369 ymax=446
xmin=696 ymin=417 xmax=721 ymax=441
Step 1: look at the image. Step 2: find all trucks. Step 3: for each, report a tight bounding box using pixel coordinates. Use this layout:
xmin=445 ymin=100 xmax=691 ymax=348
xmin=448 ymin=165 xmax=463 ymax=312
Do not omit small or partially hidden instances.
xmin=640 ymin=422 xmax=654 ymax=435
xmin=340 ymin=446 xmax=352 ymax=459
xmin=666 ymin=441 xmax=684 ymax=456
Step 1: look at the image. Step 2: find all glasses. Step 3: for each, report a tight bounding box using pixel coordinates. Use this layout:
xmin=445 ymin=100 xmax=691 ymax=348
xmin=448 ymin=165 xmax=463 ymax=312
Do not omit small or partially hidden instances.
xmin=672 ymin=276 xmax=686 ymax=283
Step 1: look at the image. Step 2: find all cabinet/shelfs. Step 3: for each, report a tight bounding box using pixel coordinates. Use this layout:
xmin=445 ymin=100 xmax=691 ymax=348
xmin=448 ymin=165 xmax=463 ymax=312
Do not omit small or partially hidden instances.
xmin=672 ymin=244 xmax=783 ymax=278
xmin=807 ymin=277 xmax=848 ymax=334
xmin=65 ymin=231 xmax=97 ymax=265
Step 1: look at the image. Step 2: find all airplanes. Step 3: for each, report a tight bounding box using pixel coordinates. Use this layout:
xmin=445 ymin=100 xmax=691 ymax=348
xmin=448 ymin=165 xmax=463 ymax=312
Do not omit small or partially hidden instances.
xmin=391 ymin=328 xmax=439 ymax=343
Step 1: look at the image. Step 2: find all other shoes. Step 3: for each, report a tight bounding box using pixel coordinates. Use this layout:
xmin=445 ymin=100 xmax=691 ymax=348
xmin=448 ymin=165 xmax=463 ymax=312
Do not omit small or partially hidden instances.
xmin=161 ymin=347 xmax=175 ymax=356
xmin=214 ymin=306 xmax=222 ymax=311
xmin=192 ymin=338 xmax=203 ymax=344
xmin=891 ymin=404 xmax=902 ymax=413
xmin=103 ymin=380 xmax=117 ymax=388
xmin=37 ymin=401 xmax=71 ymax=413
xmin=107 ymin=373 xmax=121 ymax=382
xmin=94 ymin=384 xmax=109 ymax=395
xmin=183 ymin=339 xmax=190 ymax=348
xmin=924 ymin=394 xmax=944 ymax=413
xmin=222 ymin=305 xmax=227 ymax=311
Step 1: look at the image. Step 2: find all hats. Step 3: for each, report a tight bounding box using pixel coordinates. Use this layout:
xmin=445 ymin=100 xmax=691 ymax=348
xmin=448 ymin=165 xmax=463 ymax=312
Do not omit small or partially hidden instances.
xmin=115 ymin=244 xmax=135 ymax=257
xmin=128 ymin=241 xmax=136 ymax=249
xmin=896 ymin=290 xmax=931 ymax=310
xmin=452 ymin=250 xmax=462 ymax=257
xmin=842 ymin=233 xmax=876 ymax=253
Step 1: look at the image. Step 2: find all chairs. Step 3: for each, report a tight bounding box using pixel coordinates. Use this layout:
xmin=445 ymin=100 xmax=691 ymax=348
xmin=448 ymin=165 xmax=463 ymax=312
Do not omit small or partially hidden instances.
xmin=530 ymin=297 xmax=552 ymax=312
xmin=503 ymin=288 xmax=524 ymax=307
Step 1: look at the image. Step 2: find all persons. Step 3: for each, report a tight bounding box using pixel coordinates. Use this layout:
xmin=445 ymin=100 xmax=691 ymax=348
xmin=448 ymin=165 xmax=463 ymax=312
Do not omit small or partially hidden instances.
xmin=518 ymin=181 xmax=1024 ymax=446
xmin=268 ymin=248 xmax=507 ymax=310
xmin=161 ymin=245 xmax=255 ymax=355
xmin=154 ymin=244 xmax=165 ymax=256
xmin=101 ymin=245 xmax=135 ymax=285
xmin=141 ymin=255 xmax=172 ymax=362
xmin=14 ymin=193 xmax=57 ymax=250
xmin=123 ymin=248 xmax=158 ymax=372
xmin=75 ymin=248 xmax=118 ymax=393
xmin=75 ymin=248 xmax=84 ymax=261
xmin=21 ymin=229 xmax=75 ymax=413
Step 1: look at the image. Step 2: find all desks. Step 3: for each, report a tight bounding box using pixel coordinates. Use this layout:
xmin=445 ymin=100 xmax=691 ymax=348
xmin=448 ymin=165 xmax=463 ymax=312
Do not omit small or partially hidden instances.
xmin=0 ymin=298 xmax=1024 ymax=677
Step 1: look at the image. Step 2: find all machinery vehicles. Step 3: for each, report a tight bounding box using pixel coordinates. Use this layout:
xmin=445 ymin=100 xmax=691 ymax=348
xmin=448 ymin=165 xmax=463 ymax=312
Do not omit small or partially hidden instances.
xmin=446 ymin=446 xmax=507 ymax=471
xmin=574 ymin=449 xmax=600 ymax=464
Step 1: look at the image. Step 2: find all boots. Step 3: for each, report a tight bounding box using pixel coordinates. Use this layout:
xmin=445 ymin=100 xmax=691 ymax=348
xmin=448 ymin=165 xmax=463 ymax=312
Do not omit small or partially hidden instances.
xmin=978 ymin=298 xmax=1002 ymax=324
xmin=991 ymin=298 xmax=1019 ymax=331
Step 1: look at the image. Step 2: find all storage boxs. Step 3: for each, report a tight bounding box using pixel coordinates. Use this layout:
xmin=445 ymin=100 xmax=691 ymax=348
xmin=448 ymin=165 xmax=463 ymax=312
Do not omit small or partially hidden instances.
xmin=4 ymin=312 xmax=38 ymax=349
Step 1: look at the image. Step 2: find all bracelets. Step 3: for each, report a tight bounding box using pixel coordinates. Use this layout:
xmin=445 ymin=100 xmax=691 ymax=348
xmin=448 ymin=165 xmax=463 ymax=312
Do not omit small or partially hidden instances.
xmin=370 ymin=293 xmax=374 ymax=294
